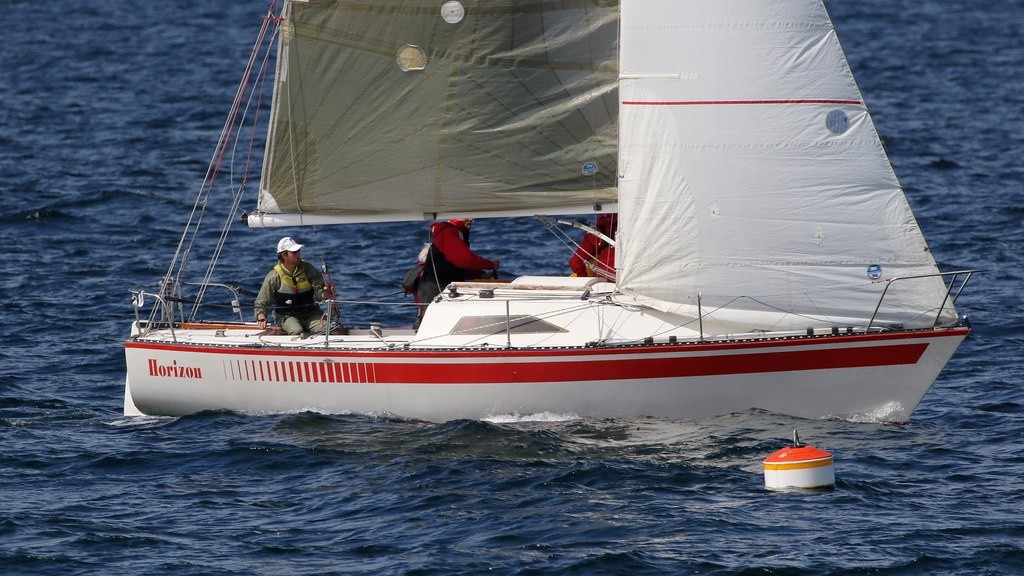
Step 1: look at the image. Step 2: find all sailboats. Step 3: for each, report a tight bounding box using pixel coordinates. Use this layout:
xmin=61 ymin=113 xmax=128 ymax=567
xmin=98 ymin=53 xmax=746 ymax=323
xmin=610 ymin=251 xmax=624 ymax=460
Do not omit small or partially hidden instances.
xmin=117 ymin=0 xmax=976 ymax=435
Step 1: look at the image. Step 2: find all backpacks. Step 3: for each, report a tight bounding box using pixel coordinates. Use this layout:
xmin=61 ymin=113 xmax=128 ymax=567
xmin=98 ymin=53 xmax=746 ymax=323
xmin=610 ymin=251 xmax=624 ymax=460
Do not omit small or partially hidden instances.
xmin=402 ymin=262 xmax=426 ymax=297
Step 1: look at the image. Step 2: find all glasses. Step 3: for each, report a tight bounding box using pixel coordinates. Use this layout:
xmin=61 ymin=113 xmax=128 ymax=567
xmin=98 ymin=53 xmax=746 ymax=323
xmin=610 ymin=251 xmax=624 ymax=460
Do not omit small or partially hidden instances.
xmin=287 ymin=249 xmax=300 ymax=255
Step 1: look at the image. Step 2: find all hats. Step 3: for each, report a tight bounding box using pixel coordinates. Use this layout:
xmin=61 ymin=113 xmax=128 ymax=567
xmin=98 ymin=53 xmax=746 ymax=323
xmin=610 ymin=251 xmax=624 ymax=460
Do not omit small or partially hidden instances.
xmin=276 ymin=237 xmax=304 ymax=253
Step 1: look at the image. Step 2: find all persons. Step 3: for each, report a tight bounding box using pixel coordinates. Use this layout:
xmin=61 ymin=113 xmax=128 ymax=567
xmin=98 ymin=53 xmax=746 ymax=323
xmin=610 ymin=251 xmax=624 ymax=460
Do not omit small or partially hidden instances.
xmin=254 ymin=237 xmax=336 ymax=336
xmin=411 ymin=218 xmax=501 ymax=320
xmin=568 ymin=213 xmax=618 ymax=278
